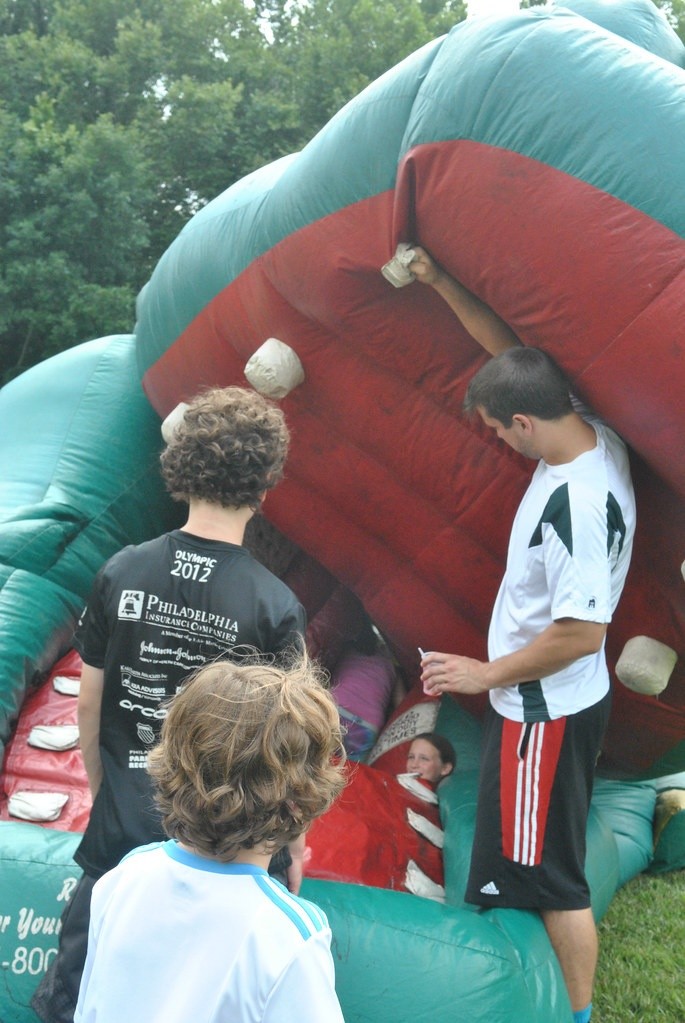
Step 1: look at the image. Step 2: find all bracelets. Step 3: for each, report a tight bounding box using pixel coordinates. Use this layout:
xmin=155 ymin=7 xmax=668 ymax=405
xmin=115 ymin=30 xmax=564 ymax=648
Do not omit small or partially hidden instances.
xmin=290 ymin=847 xmax=312 ymax=862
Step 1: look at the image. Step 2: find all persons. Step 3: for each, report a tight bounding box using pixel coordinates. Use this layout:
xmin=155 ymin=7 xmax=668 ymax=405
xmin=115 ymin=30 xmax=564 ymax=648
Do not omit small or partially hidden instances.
xmin=330 ymin=622 xmax=406 ymax=766
xmin=29 ymin=385 xmax=313 ymax=1023
xmin=70 ymin=661 xmax=346 ymax=1023
xmin=399 ymin=243 xmax=635 ymax=1023
xmin=405 ymin=734 xmax=456 ymax=782
